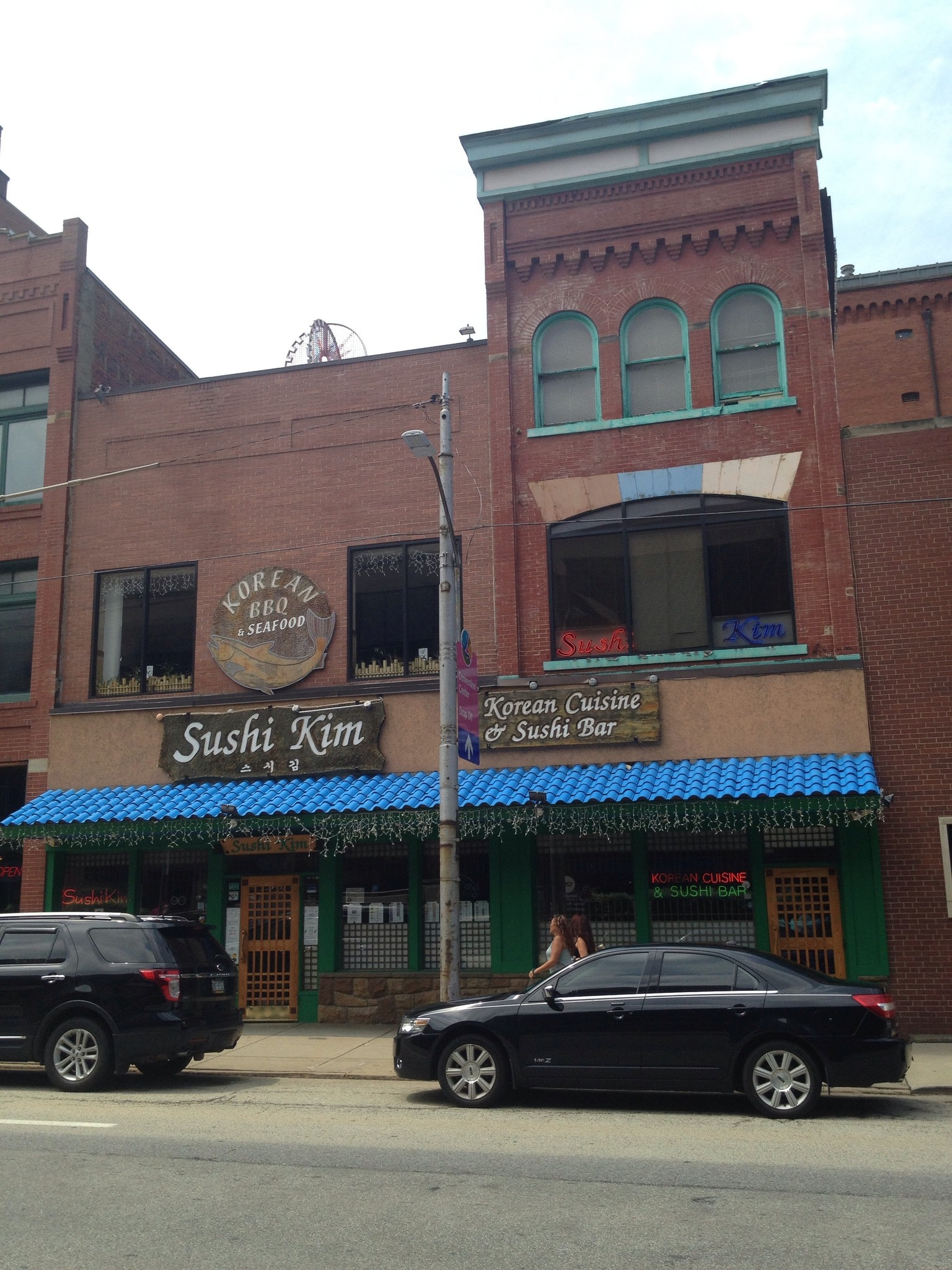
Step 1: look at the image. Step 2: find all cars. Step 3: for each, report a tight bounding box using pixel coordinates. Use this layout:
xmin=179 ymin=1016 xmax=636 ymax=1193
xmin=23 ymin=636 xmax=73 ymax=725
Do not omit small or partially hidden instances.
xmin=392 ymin=941 xmax=912 ymax=1118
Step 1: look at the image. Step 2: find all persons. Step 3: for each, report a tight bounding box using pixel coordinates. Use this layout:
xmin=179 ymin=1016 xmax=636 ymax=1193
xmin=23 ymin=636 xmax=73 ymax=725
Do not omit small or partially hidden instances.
xmin=570 ymin=913 xmax=594 ymax=964
xmin=528 ymin=914 xmax=574 ymax=983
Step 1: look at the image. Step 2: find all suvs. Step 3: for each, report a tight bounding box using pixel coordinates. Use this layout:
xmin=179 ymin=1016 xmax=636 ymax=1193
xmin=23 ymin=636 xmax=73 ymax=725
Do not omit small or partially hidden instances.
xmin=0 ymin=911 xmax=245 ymax=1092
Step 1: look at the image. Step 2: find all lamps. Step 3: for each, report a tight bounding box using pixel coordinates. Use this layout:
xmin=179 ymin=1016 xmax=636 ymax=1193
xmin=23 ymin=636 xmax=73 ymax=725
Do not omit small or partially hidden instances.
xmin=43 ymin=836 xmax=61 ymax=847
xmin=459 ymin=324 xmax=476 ymax=342
xmin=94 ymin=385 xmax=111 ymax=403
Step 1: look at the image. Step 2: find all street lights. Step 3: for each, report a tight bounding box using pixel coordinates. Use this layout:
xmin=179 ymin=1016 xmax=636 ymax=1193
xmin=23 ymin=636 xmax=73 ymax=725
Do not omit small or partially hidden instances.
xmin=403 ymin=370 xmax=464 ymax=1002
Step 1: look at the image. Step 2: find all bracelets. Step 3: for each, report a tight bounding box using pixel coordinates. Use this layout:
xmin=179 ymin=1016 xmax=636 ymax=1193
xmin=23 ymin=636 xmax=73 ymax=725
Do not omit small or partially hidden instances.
xmin=532 ymin=968 xmax=539 ymax=976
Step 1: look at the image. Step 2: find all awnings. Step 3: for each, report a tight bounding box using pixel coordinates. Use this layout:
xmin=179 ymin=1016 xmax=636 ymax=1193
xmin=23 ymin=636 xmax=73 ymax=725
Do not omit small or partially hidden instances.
xmin=0 ymin=752 xmax=890 ymax=858
